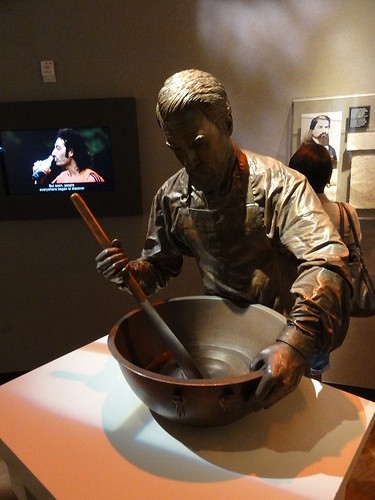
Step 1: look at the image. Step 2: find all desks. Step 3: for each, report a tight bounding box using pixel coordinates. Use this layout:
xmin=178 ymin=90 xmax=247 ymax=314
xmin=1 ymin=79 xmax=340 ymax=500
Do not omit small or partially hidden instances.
xmin=0 ymin=335 xmax=375 ymax=500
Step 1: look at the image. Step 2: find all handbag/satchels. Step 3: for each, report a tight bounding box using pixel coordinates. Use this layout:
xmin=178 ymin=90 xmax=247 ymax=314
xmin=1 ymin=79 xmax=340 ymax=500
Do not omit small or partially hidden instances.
xmin=335 ymin=201 xmax=375 ymax=316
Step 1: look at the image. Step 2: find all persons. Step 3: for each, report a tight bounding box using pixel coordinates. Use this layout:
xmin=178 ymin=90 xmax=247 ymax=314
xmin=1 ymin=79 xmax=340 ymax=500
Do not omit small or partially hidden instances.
xmin=289 ymin=144 xmax=362 ymax=277
xmin=94 ymin=70 xmax=355 ymax=411
xmin=32 ymin=129 xmax=106 ymax=182
xmin=309 ymin=115 xmax=337 ymax=168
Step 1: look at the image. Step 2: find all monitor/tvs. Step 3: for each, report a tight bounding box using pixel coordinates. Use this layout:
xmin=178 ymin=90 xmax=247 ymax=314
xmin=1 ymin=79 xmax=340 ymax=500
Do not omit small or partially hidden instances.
xmin=0 ymin=97 xmax=144 ymax=220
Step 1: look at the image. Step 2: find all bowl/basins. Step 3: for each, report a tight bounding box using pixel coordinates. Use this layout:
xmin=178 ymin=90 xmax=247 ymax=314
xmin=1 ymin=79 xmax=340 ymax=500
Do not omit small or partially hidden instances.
xmin=107 ymin=295 xmax=304 ymax=427
